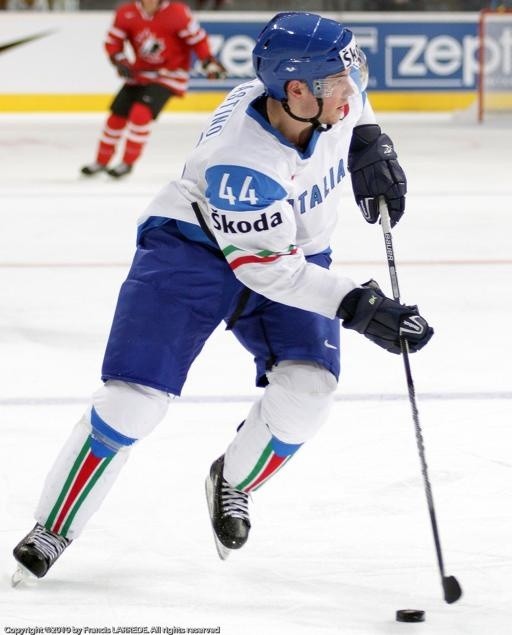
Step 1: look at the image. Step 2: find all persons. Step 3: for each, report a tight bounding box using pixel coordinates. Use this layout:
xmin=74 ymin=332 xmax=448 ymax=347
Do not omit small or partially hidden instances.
xmin=11 ymin=11 xmax=435 ymax=579
xmin=82 ymin=1 xmax=228 ymax=179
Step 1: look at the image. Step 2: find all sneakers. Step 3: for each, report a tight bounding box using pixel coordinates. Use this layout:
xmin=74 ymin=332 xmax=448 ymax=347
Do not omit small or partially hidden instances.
xmin=80 ymin=162 xmax=103 ymax=174
xmin=13 ymin=522 xmax=73 ymax=578
xmin=210 ymin=454 xmax=251 ymax=550
xmin=108 ymin=162 xmax=132 ymax=176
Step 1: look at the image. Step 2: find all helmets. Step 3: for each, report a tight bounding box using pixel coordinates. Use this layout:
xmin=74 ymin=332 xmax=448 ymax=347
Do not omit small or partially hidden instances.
xmin=252 ymin=12 xmax=369 ymax=103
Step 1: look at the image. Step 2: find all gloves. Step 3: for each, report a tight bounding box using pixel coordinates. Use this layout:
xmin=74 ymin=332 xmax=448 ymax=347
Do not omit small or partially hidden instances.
xmin=335 ymin=279 xmax=433 ymax=355
xmin=201 ymin=55 xmax=225 ymax=82
xmin=112 ymin=50 xmax=137 ymax=79
xmin=348 ymin=124 xmax=406 ymax=229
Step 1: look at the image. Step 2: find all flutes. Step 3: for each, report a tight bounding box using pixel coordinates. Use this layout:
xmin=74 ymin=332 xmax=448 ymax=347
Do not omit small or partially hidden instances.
xmin=376 ymin=194 xmax=462 ymax=603
